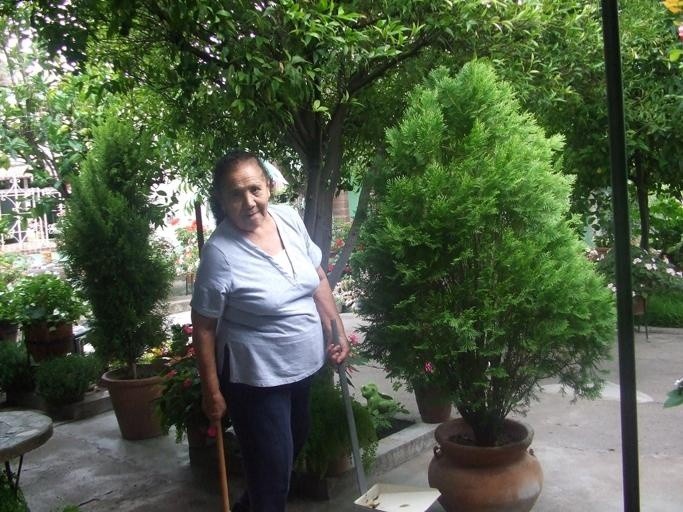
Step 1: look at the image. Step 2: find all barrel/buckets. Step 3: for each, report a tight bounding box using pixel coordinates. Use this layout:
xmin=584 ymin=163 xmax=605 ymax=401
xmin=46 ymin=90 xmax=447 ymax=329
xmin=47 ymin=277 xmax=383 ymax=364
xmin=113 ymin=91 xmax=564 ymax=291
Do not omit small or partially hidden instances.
xmin=21 ymin=316 xmax=75 ymax=363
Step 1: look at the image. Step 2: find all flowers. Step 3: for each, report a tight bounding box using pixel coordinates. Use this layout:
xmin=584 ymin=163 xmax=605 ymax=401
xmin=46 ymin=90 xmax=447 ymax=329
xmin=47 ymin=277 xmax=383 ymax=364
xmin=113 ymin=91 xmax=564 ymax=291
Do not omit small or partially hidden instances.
xmin=147 ymin=323 xmax=256 ymax=442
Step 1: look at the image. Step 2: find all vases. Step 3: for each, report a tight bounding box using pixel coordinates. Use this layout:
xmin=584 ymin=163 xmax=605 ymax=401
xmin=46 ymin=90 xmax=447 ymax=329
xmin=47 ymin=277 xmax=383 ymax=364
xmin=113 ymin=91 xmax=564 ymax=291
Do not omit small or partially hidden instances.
xmin=188 ymin=425 xmax=219 ymax=478
xmin=411 ymin=367 xmax=454 ymax=425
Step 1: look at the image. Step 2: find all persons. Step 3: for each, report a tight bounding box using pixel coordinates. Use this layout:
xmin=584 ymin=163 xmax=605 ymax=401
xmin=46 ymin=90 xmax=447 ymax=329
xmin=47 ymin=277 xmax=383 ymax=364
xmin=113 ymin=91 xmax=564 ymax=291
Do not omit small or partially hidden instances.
xmin=192 ymin=150 xmax=350 ymax=512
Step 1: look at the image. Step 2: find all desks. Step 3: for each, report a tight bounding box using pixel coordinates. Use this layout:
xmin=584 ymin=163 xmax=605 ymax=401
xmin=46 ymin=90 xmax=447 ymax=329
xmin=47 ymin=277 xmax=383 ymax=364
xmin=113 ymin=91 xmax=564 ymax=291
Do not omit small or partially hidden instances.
xmin=1 ymin=411 xmax=53 ymax=505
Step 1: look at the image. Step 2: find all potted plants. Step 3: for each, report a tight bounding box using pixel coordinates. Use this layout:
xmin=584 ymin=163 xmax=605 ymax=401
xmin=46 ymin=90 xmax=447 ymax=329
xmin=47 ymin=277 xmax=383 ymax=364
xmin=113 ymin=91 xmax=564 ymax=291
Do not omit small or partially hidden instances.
xmin=0 ymin=265 xmax=89 ymax=363
xmin=54 ymin=114 xmax=177 ymax=440
xmin=354 ymin=57 xmax=621 ymax=512
